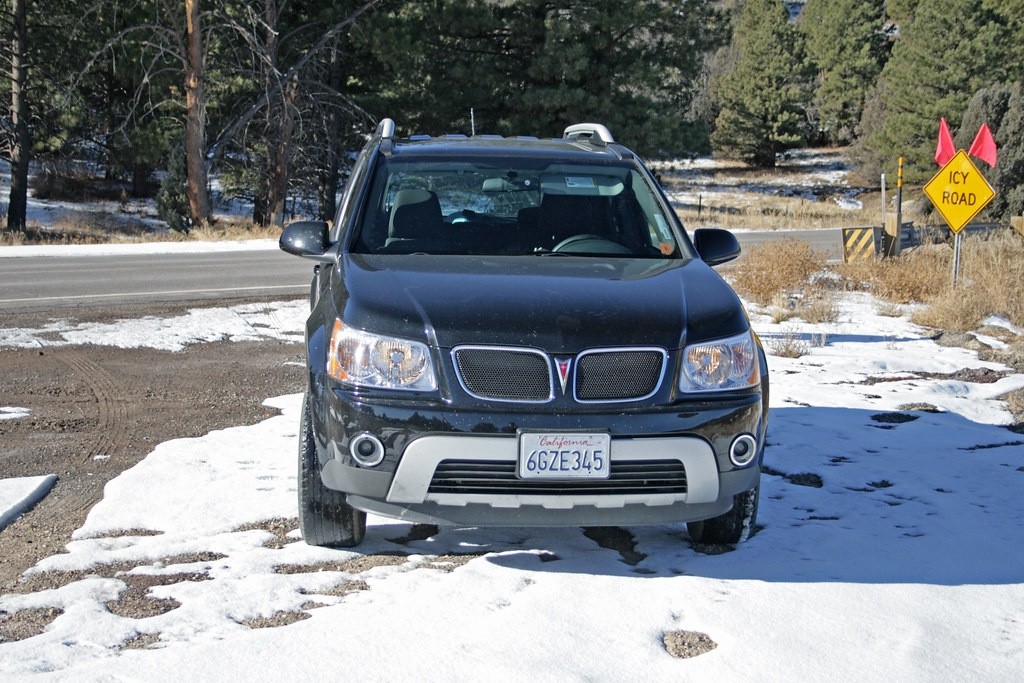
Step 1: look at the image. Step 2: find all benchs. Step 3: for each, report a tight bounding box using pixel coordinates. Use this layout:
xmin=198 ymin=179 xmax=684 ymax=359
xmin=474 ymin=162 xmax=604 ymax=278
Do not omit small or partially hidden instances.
xmin=444 ymin=208 xmax=540 ymax=247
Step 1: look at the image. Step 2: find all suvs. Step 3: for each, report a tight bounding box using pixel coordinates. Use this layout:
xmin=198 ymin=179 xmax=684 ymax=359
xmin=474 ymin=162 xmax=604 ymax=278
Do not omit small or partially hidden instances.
xmin=271 ymin=118 xmax=770 ymax=560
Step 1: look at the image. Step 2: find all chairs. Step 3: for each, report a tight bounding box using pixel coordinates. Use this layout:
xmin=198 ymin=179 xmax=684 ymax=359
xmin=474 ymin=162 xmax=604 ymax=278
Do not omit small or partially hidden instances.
xmin=385 ymin=190 xmax=443 ymax=254
xmin=538 ymin=192 xmax=620 ymax=250
